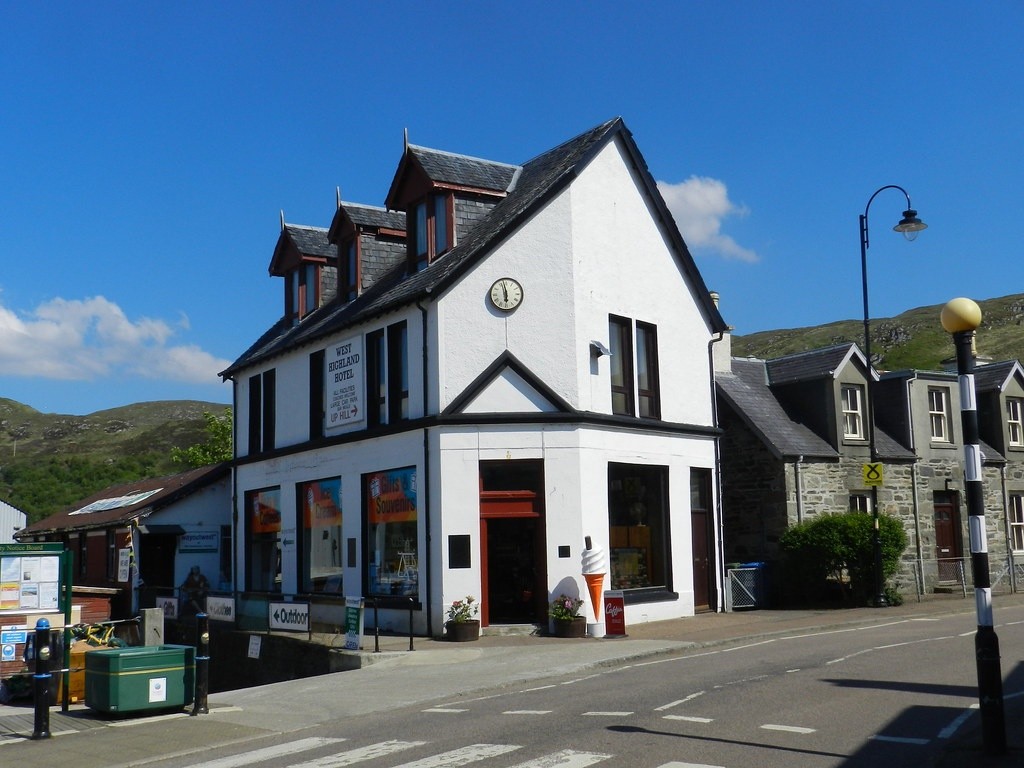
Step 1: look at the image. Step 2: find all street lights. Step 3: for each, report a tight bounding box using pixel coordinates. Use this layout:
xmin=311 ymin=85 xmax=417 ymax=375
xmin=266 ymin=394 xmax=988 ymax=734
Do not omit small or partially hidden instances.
xmin=851 ymin=184 xmax=923 ymax=608
xmin=940 ymin=296 xmax=1008 ymax=754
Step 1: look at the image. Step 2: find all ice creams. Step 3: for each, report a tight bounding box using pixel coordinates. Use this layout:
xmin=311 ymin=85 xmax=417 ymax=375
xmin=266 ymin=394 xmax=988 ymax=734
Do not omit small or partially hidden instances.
xmin=581 ymin=535 xmax=607 ymax=622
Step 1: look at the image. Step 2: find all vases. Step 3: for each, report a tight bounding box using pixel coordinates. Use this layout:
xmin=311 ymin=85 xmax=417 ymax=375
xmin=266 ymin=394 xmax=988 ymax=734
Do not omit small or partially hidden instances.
xmin=446 ymin=619 xmax=480 ymax=641
xmin=554 ymin=615 xmax=586 ymax=638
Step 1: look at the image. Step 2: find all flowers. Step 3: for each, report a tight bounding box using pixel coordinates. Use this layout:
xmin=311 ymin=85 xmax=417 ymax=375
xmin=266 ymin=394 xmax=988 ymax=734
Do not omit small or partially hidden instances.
xmin=546 ymin=594 xmax=585 ymax=621
xmin=444 ymin=595 xmax=479 ymax=622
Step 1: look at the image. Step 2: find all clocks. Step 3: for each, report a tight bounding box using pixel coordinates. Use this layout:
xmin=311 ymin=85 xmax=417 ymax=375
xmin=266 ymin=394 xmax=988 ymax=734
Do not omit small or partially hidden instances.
xmin=489 ymin=277 xmax=525 ymax=312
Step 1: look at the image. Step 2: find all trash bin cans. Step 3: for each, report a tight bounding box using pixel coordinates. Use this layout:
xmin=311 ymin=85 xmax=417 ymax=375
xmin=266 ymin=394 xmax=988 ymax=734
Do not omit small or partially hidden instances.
xmin=84 ymin=645 xmax=196 ymax=719
